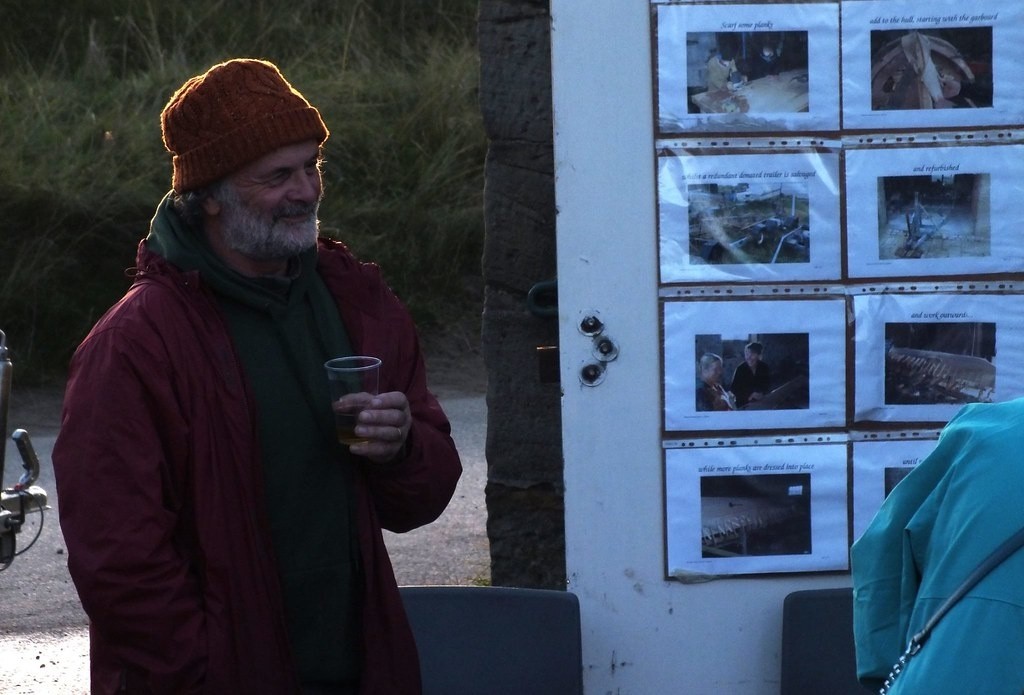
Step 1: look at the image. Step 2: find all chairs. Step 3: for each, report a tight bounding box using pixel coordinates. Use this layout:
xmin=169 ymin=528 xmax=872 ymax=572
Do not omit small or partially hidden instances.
xmin=782 ymin=588 xmax=890 ymax=695
xmin=399 ymin=586 xmax=584 ymax=695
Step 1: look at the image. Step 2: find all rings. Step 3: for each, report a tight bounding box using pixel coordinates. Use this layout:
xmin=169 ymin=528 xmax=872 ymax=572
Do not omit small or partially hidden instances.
xmin=397 ymin=428 xmax=402 ymax=438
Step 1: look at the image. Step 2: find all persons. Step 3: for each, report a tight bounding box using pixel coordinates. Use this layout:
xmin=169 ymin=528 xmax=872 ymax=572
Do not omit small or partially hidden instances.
xmin=707 ymin=52 xmax=748 ymax=91
xmin=52 ymin=58 xmax=463 ymax=695
xmin=696 ymin=353 xmax=736 ymax=411
xmin=849 ymin=397 xmax=1024 ymax=695
xmin=730 ymin=343 xmax=776 ymax=410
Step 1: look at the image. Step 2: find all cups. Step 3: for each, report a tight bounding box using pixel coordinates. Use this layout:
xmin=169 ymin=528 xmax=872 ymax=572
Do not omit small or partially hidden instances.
xmin=326 ymin=357 xmax=380 ymax=445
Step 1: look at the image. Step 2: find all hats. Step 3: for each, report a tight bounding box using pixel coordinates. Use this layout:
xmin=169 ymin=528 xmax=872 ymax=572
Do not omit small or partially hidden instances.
xmin=160 ymin=58 xmax=328 ymax=193
